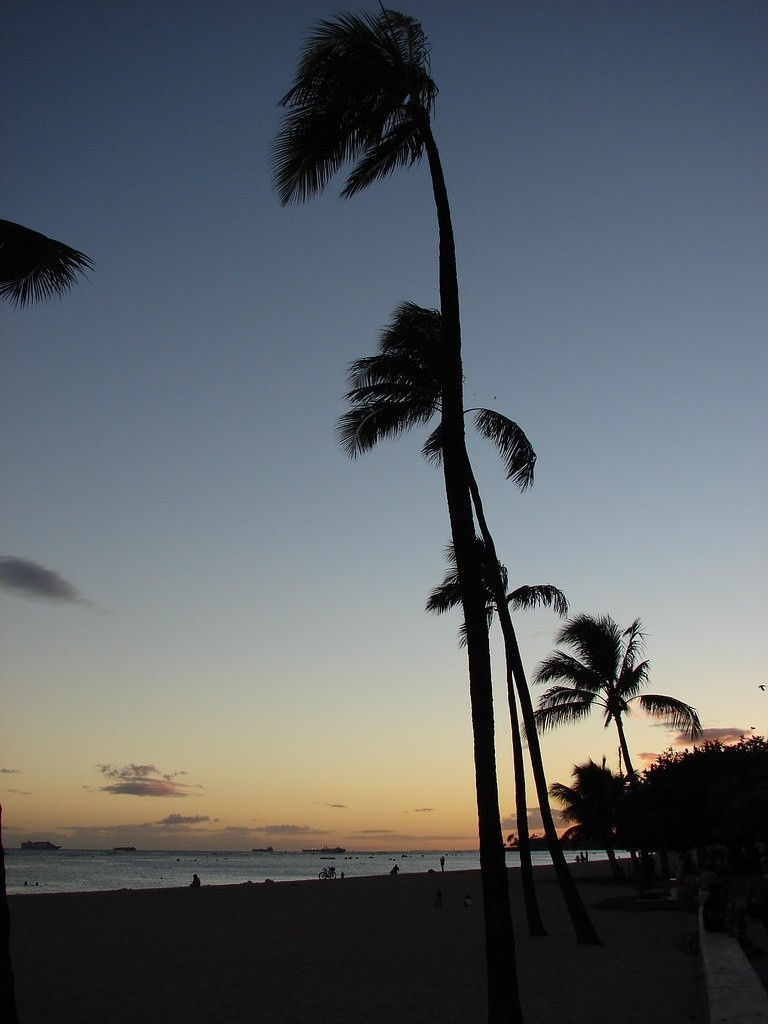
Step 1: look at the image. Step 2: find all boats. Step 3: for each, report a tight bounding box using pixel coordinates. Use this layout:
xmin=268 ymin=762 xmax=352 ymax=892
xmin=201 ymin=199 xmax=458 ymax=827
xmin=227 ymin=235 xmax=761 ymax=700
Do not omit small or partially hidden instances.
xmin=253 ymin=847 xmax=273 ymax=852
xmin=321 ymin=857 xmax=335 ymax=859
xmin=113 ymin=846 xmax=137 ymax=852
xmin=302 ymin=847 xmax=345 ymax=854
xmin=22 ymin=841 xmax=62 ymax=851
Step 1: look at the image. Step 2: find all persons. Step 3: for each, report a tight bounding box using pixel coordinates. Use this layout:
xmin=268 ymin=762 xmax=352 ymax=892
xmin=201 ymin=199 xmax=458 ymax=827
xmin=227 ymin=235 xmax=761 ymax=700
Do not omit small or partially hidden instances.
xmin=433 ymin=888 xmax=443 ymax=910
xmin=393 ymin=865 xmax=399 ymax=874
xmin=703 ymin=855 xmax=768 ymax=959
xmin=632 ymin=850 xmax=702 ymax=895
xmin=190 ymin=874 xmax=200 ymax=889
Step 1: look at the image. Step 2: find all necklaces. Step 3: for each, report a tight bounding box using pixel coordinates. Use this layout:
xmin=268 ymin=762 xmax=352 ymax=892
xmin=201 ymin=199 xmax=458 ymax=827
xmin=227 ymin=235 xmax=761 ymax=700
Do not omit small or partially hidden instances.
xmin=463 ymin=894 xmax=473 ymax=909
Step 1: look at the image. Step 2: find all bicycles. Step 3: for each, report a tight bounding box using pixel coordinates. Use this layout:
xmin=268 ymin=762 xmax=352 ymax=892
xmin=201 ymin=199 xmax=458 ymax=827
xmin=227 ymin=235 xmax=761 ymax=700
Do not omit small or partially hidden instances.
xmin=318 ymin=867 xmax=336 ymax=880
